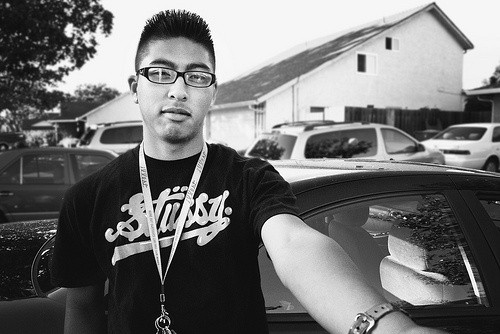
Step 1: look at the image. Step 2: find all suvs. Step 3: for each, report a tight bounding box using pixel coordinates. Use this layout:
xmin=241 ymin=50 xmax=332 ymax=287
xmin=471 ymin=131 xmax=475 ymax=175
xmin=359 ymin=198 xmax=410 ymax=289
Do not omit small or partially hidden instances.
xmin=74 ymin=119 xmax=144 ymax=167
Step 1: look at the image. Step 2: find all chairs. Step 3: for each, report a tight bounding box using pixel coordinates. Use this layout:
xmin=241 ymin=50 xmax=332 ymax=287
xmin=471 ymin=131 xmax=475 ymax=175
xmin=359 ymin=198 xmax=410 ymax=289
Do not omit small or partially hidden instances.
xmin=379 ymin=218 xmax=455 ymax=307
xmin=328 ymin=204 xmax=385 ymax=296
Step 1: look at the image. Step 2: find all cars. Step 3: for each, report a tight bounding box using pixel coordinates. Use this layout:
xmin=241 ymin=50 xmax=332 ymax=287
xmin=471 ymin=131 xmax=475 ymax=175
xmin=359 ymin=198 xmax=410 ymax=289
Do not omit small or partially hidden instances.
xmin=0 ymin=148 xmax=122 ymax=223
xmin=420 ymin=122 xmax=500 ymax=171
xmin=1 ymin=158 xmax=500 ymax=334
xmin=243 ymin=119 xmax=447 ymax=165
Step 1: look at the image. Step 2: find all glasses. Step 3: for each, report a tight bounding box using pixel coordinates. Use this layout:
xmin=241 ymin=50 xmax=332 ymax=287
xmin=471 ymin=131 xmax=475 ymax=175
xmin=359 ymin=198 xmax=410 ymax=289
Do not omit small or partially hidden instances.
xmin=135 ymin=67 xmax=217 ymax=89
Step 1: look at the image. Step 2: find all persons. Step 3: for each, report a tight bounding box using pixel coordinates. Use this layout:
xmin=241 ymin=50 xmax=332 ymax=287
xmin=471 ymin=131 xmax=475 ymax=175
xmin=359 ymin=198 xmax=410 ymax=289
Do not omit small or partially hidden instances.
xmin=51 ymin=9 xmax=450 ymax=334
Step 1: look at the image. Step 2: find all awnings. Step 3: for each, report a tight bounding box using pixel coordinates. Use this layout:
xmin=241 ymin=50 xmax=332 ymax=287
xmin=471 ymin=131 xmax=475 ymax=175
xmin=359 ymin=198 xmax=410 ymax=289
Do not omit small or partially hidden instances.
xmin=22 ymin=117 xmax=54 ymax=130
xmin=46 ymin=117 xmax=87 ymax=127
xmin=461 ymin=86 xmax=500 ymax=99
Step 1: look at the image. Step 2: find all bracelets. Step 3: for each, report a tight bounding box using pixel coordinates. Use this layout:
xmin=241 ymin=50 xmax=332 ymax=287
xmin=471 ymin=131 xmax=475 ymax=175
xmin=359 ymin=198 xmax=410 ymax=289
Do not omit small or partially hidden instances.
xmin=342 ymin=302 xmax=408 ymax=334
